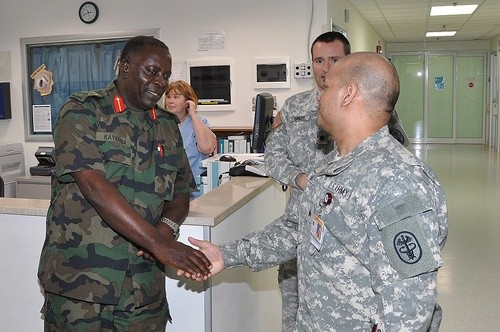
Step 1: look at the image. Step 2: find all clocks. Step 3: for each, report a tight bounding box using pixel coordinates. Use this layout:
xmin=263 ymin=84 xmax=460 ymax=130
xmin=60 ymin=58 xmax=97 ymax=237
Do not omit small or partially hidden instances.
xmin=79 ymin=1 xmax=99 ymax=24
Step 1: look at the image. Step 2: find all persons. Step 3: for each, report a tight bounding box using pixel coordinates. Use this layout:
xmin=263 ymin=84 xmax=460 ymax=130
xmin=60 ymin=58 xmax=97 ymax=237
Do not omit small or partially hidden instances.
xmin=164 ymin=80 xmax=217 ymax=201
xmin=176 ymin=52 xmax=448 ymax=332
xmin=38 ymin=36 xmax=213 ymax=332
xmin=264 ymin=32 xmax=410 ymax=332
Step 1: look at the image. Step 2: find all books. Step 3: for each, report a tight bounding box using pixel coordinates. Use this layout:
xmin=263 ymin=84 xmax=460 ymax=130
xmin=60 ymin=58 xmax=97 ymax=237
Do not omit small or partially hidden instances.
xmin=216 ymin=134 xmax=253 ymax=154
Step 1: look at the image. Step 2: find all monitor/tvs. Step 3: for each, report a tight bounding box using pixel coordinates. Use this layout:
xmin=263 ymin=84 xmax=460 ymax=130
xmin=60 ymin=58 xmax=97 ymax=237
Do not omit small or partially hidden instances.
xmin=0 ymin=82 xmax=12 ymax=120
xmin=252 ymin=93 xmax=276 ymax=150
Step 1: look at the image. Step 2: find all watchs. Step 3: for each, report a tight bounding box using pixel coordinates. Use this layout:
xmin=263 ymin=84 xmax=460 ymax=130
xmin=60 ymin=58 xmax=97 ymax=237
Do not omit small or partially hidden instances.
xmin=159 ymin=217 xmax=180 ymax=241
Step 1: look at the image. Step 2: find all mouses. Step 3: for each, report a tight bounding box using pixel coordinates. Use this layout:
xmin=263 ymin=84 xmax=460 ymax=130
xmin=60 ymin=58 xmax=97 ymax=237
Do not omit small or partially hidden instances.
xmin=220 ymin=155 xmax=236 ymax=161
